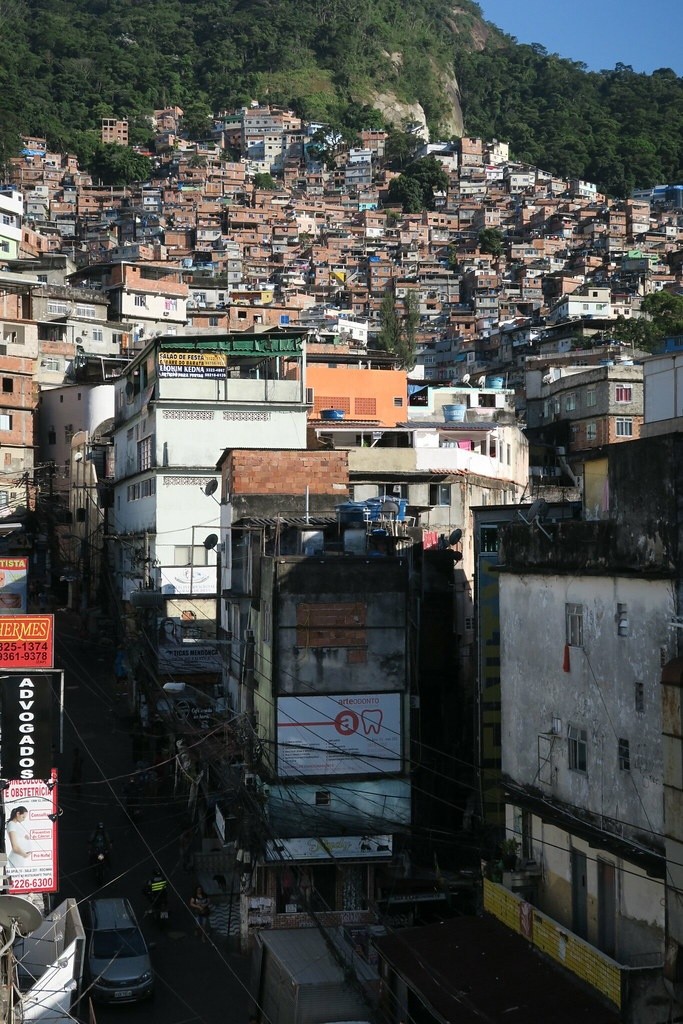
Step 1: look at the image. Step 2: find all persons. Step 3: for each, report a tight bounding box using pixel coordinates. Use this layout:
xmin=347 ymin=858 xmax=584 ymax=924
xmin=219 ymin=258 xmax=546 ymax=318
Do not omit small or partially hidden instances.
xmin=190 ymin=885 xmax=209 ymax=944
xmin=88 ymin=823 xmax=112 ymax=853
xmin=145 ymin=868 xmax=169 ymax=893
xmin=159 ymin=618 xmax=178 ymax=644
xmin=123 ymin=777 xmax=140 ymax=806
xmin=7 ymin=806 xmax=30 ymax=867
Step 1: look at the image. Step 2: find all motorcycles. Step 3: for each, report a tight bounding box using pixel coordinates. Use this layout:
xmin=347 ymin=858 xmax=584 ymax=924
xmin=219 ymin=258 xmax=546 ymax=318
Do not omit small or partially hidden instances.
xmin=144 ymin=888 xmax=167 ymax=930
xmin=122 ymin=783 xmax=143 ymax=824
xmin=85 ymin=834 xmax=113 ymax=880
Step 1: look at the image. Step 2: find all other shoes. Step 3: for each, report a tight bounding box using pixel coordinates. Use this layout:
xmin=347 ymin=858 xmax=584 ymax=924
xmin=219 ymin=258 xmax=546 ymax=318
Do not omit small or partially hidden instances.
xmin=195 ymin=930 xmax=197 ymax=935
xmin=202 ymin=936 xmax=206 ymax=943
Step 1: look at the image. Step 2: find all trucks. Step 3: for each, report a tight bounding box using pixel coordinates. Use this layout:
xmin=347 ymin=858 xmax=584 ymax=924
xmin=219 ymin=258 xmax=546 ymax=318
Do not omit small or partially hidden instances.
xmin=248 ymin=928 xmax=381 ymax=1024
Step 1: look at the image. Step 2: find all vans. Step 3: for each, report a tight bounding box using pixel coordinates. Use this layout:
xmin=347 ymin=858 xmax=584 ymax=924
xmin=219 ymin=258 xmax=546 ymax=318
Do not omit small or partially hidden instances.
xmin=83 ymin=898 xmax=153 ymax=1006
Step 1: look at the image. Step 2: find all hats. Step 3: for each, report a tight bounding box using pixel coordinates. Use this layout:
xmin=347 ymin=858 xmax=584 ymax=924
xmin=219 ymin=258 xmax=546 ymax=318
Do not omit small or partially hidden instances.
xmin=99 ymin=822 xmax=104 ymax=828
xmin=153 ymin=867 xmax=161 ymax=874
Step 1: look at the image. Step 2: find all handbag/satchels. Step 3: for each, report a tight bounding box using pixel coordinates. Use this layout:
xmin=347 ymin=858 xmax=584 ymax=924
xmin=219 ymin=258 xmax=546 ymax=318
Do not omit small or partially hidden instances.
xmin=200 ymin=898 xmax=210 ymax=915
